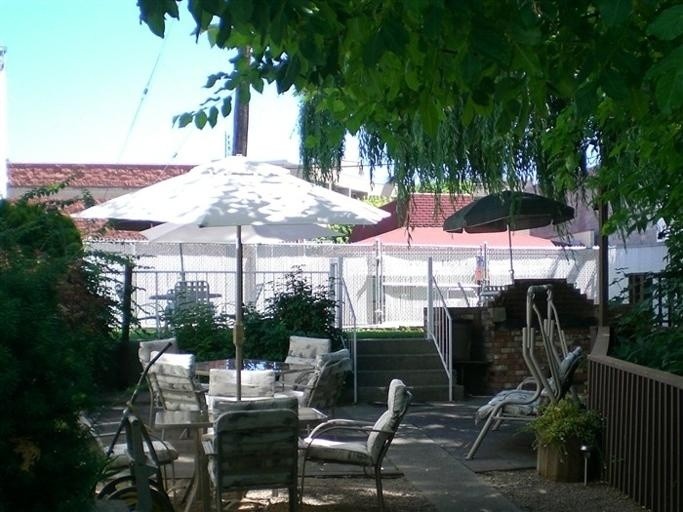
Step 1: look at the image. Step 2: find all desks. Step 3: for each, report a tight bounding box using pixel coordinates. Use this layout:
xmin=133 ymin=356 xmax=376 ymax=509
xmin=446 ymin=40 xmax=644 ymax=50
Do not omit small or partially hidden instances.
xmin=150 ymin=294 xmax=222 ymax=300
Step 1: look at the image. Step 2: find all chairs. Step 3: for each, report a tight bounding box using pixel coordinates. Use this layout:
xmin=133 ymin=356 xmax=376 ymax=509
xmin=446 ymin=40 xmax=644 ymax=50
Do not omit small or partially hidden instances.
xmin=115 ymin=281 xmax=209 ymax=332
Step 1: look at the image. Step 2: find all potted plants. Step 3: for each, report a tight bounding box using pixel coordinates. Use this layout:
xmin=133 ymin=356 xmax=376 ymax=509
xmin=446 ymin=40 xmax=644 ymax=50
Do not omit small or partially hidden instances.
xmin=527 ymin=387 xmax=607 ymax=483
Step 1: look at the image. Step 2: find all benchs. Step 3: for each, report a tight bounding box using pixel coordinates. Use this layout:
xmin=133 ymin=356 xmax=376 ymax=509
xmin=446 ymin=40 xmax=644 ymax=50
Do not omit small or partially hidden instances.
xmin=465 ymin=285 xmax=586 ymax=460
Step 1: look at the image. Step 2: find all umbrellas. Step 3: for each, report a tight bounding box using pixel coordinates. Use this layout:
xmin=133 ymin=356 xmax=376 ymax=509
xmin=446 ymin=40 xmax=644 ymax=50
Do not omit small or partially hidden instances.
xmin=443 ymin=188 xmax=575 ymax=283
xmin=136 ymin=218 xmax=348 ymax=246
xmin=67 ymin=151 xmax=395 ymax=399
